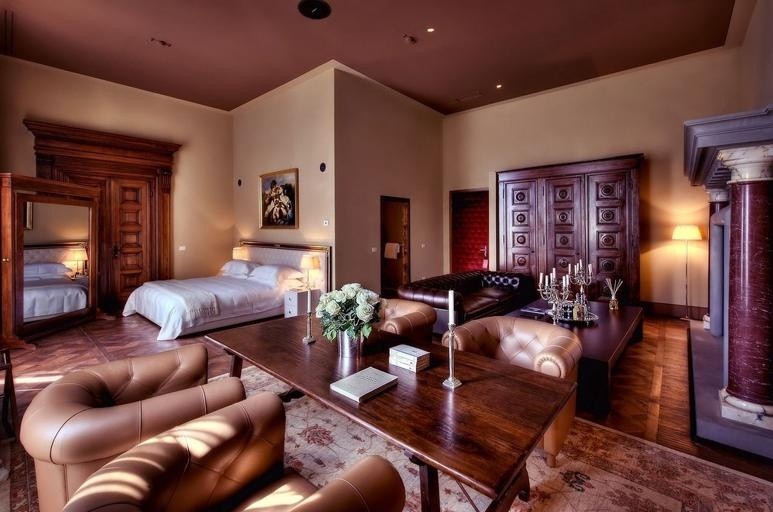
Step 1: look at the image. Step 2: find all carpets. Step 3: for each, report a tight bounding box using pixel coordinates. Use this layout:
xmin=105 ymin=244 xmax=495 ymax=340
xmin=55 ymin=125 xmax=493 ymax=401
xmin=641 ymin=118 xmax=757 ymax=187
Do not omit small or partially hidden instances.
xmin=1 ymin=365 xmax=773 ymax=512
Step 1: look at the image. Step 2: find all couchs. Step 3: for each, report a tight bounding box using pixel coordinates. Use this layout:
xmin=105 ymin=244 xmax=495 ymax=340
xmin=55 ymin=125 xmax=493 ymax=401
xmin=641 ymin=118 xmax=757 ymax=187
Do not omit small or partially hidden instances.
xmin=398 ymin=270 xmax=536 ymax=326
xmin=19 ymin=342 xmax=248 ymax=512
xmin=442 ymin=315 xmax=583 ymax=469
xmin=378 ymin=298 xmax=438 ymax=344
xmin=62 ymin=392 xmax=406 ymax=512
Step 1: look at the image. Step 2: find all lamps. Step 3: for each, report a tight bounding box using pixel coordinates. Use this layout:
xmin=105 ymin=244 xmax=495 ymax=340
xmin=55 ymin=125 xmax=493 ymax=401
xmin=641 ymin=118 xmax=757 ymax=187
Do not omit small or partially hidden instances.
xmin=67 ymin=249 xmax=88 ymax=278
xmin=672 ymin=224 xmax=702 ymax=323
xmin=233 ymin=246 xmax=249 ymax=262
xmin=301 ymin=253 xmax=321 ymax=289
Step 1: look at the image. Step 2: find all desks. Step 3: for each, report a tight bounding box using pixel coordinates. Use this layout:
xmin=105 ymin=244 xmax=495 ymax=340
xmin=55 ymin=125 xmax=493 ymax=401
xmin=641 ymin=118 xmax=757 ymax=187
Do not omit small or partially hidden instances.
xmin=204 ymin=311 xmax=578 ymax=512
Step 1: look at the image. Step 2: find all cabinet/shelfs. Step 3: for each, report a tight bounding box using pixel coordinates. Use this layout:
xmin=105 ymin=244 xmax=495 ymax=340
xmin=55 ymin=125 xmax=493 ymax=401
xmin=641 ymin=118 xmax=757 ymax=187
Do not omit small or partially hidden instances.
xmin=542 ymin=162 xmax=584 ymax=300
xmin=496 ymin=166 xmax=542 ymax=285
xmin=584 ymin=153 xmax=641 ymax=304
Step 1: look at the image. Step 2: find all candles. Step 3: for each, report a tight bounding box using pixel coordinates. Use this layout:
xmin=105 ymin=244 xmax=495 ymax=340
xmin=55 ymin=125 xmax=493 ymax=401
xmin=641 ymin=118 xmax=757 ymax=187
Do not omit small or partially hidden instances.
xmin=575 ymin=265 xmax=577 ymax=275
xmin=589 ymin=263 xmax=593 ymax=275
xmin=447 ymin=289 xmax=456 ymax=326
xmin=550 ymin=273 xmax=553 ymax=284
xmin=569 ymin=264 xmax=572 ymax=273
xmin=577 ymin=263 xmax=579 ymax=272
xmin=563 ymin=276 xmax=566 ymax=287
xmin=307 ymin=288 xmax=312 ymax=313
xmin=553 ymin=268 xmax=555 ymax=278
xmin=539 ymin=271 xmax=544 ymax=284
xmin=544 ymin=275 xmax=549 ymax=287
xmin=580 ymin=258 xmax=583 ymax=268
xmin=566 ymin=275 xmax=569 ymax=286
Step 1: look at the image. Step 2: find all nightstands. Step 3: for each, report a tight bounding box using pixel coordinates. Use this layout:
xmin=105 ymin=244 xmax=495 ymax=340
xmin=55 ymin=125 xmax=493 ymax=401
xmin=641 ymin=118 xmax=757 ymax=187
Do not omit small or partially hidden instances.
xmin=284 ymin=288 xmax=320 ymax=319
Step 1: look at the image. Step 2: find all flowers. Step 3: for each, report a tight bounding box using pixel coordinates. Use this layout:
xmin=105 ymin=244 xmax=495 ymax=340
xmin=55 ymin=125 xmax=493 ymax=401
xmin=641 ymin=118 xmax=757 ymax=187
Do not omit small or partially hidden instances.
xmin=315 ymin=283 xmax=389 ymax=343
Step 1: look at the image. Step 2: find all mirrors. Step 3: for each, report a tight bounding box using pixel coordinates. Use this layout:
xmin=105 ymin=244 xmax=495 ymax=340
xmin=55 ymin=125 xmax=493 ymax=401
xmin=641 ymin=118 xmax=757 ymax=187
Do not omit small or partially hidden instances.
xmin=22 ymin=200 xmax=93 ymax=323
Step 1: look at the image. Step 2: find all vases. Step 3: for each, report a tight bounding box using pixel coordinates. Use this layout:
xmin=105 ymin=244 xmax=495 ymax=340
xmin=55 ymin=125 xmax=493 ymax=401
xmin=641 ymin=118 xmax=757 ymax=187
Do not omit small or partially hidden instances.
xmin=336 ymin=331 xmax=362 ymax=358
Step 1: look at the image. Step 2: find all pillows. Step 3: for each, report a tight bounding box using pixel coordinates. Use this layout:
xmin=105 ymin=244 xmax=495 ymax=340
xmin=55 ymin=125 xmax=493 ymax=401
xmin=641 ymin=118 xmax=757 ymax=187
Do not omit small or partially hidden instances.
xmin=248 ymin=277 xmax=305 ymax=289
xmin=40 ymin=263 xmax=72 ymax=274
xmin=42 ymin=275 xmax=66 ymax=280
xmin=220 ymin=260 xmax=261 ymax=275
xmin=249 ymin=264 xmax=305 ymax=280
xmin=24 ymin=277 xmax=42 ymax=282
xmin=217 ymin=272 xmax=251 ymax=279
xmin=24 ymin=263 xmax=41 ymax=276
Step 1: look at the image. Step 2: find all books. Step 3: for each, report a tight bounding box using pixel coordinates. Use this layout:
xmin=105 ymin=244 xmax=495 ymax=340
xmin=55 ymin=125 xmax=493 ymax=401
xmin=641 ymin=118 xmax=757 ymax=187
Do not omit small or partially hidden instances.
xmin=330 ymin=367 xmax=397 ymax=403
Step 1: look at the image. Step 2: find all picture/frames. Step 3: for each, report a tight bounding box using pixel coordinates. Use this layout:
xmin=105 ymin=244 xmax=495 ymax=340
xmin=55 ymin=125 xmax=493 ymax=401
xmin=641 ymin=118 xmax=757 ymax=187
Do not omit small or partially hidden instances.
xmin=259 ymin=168 xmax=300 ymax=230
xmin=23 ymin=203 xmax=33 ymax=231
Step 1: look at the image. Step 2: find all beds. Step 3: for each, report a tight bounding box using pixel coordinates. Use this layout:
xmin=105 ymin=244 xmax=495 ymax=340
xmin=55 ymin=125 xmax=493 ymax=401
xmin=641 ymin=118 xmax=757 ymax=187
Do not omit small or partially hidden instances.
xmin=122 ymin=239 xmax=332 ymax=341
xmin=23 ymin=240 xmax=87 ymax=318
